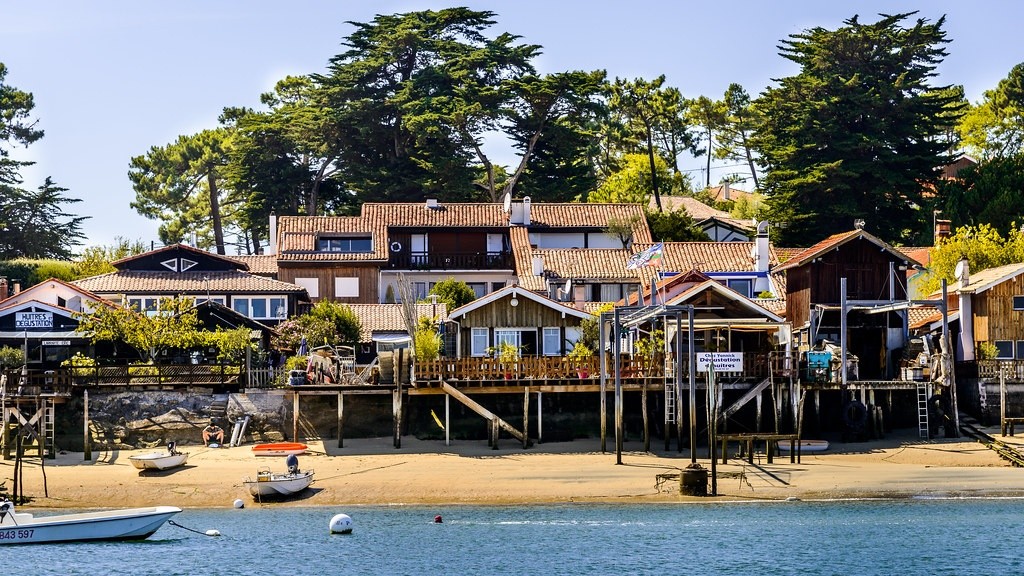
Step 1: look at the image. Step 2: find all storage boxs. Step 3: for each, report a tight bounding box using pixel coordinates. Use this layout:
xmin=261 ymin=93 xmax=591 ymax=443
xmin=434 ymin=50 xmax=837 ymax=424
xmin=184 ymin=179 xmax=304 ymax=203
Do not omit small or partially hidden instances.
xmin=287 ymin=370 xmax=308 ymax=386
xmin=901 ymin=366 xmax=923 ymax=381
xmin=914 ymin=352 xmax=930 ymax=381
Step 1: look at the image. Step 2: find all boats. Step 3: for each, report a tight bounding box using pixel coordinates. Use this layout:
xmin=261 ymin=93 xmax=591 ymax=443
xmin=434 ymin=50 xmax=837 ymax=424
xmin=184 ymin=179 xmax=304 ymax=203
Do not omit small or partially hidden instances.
xmin=0 ymin=500 xmax=183 ymax=543
xmin=127 ymin=450 xmax=190 ymax=471
xmin=243 ymin=464 xmax=315 ymax=500
xmin=251 ymin=443 xmax=308 ymax=456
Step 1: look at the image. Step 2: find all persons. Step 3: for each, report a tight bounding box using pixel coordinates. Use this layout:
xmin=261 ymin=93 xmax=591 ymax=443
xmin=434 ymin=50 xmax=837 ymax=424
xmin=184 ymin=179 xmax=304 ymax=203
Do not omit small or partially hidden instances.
xmin=202 ymin=422 xmax=225 ymax=447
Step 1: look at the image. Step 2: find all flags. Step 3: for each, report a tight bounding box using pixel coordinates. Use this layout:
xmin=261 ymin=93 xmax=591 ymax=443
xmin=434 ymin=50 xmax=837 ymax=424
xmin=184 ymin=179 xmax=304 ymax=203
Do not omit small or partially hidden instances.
xmin=625 ymin=243 xmax=663 ymax=271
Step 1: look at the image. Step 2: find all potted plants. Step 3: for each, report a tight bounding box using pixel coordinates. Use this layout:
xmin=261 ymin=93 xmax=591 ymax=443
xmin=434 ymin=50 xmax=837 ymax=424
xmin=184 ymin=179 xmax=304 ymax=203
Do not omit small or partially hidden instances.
xmin=500 ymin=343 xmax=519 ymax=380
xmin=60 ymin=351 xmax=101 ymax=385
xmin=569 ymin=343 xmax=594 ymax=378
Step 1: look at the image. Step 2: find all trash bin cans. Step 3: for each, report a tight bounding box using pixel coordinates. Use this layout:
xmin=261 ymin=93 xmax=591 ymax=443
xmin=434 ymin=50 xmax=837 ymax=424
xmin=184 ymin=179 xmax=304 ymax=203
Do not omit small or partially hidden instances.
xmin=900 ymin=366 xmax=923 ymax=381
